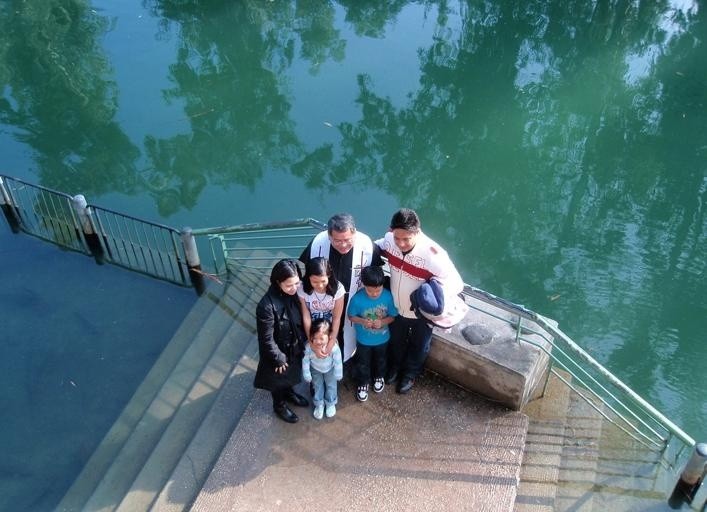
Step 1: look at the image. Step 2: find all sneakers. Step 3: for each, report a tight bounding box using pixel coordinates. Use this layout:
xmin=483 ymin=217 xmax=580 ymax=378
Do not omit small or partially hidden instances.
xmin=373 ymin=376 xmax=384 ymax=393
xmin=313 ymin=403 xmax=336 ymax=419
xmin=356 ymin=384 xmax=369 ymax=401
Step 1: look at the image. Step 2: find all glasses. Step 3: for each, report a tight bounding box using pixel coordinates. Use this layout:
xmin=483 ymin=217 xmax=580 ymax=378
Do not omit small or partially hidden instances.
xmin=330 ymin=238 xmax=353 ymax=246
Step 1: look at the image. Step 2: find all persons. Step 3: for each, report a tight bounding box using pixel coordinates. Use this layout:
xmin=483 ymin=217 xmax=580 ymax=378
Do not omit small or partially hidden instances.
xmin=369 ymin=209 xmax=464 ymax=393
xmin=294 ymin=214 xmax=374 ymax=390
xmin=253 ymin=258 xmax=347 ymax=421
xmin=348 ymin=266 xmax=399 ymax=402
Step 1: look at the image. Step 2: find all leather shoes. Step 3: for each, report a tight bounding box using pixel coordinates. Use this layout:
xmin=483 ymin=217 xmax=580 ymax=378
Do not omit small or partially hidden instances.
xmin=386 ymin=370 xmax=415 ymax=393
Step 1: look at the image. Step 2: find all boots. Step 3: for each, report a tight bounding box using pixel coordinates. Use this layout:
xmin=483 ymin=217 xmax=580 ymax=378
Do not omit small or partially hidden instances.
xmin=271 ymin=387 xmax=309 ymax=423
